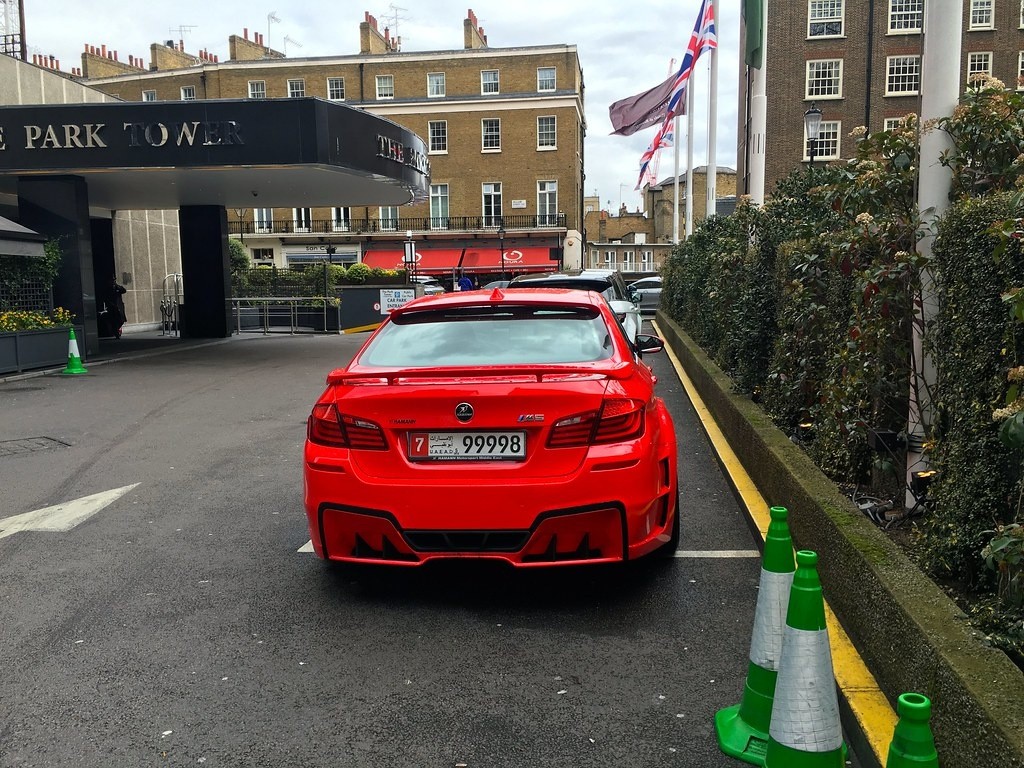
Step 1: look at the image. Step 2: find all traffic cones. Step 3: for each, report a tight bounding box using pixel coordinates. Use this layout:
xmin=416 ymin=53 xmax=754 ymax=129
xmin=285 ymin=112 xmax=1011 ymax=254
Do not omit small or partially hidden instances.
xmin=762 ymin=544 xmax=849 ymax=768
xmin=714 ymin=504 xmax=795 ymax=768
xmin=889 ymin=688 xmax=939 ymax=766
xmin=60 ymin=326 xmax=90 ymax=376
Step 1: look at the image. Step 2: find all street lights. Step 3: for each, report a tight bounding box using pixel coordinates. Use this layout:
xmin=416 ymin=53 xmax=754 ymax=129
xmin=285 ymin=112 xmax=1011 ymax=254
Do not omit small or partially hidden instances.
xmin=496 ymin=226 xmax=506 ymax=281
xmin=803 ymin=98 xmax=824 ymax=192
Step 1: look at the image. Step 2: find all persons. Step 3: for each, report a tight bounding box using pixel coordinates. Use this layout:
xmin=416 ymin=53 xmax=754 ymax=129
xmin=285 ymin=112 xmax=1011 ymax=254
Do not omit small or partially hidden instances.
xmin=104 ymin=274 xmax=125 ymax=337
xmin=458 ymin=274 xmax=472 ymax=290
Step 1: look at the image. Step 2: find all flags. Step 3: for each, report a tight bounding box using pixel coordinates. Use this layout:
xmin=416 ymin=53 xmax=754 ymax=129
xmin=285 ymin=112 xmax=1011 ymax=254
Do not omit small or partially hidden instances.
xmin=608 ymin=1 xmax=718 ymax=192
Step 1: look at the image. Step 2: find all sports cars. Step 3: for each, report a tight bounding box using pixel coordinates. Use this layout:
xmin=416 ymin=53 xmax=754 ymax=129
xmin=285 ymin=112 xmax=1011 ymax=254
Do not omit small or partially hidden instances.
xmin=298 ymin=285 xmax=684 ymax=588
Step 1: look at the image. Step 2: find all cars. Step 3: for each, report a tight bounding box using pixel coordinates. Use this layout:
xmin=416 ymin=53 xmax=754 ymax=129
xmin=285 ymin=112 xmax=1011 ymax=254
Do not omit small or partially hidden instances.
xmin=505 ymin=267 xmax=642 ymax=359
xmin=626 ymin=276 xmax=664 ymax=316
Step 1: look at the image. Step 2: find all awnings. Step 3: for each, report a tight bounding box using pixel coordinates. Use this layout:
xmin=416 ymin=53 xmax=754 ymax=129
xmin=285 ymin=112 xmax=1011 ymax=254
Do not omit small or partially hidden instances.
xmin=361 ymin=249 xmax=557 ymax=274
